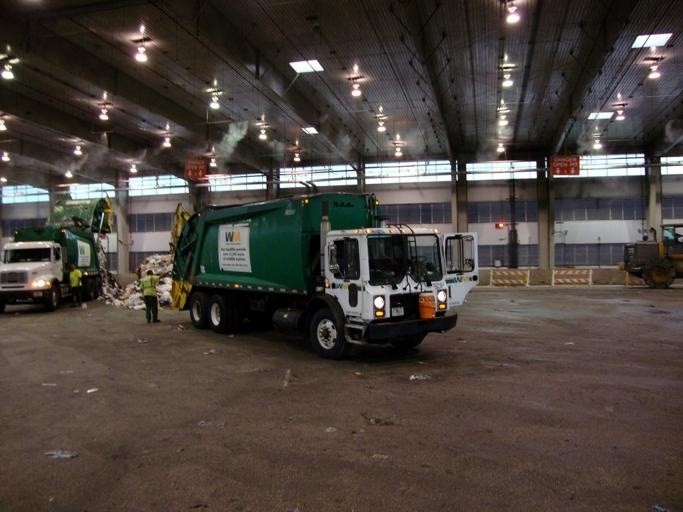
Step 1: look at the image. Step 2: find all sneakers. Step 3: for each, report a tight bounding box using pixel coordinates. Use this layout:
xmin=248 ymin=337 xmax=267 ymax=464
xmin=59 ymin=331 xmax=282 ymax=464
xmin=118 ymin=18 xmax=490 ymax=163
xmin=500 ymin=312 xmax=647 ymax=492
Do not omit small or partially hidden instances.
xmin=151 ymin=319 xmax=161 ymax=323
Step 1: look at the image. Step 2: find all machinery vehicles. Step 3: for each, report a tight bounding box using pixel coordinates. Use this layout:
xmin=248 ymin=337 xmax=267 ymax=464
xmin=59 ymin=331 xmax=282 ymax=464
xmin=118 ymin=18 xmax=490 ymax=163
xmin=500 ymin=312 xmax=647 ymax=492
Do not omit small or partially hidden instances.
xmin=623 ymin=224 xmax=683 ymax=289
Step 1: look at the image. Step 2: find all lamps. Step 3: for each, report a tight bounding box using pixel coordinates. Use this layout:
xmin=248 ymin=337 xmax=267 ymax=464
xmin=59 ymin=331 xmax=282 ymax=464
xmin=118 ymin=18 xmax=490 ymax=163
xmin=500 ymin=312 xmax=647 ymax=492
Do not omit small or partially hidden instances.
xmin=98 ymin=104 xmax=109 ymax=121
xmin=648 ymin=63 xmax=660 ymax=79
xmin=209 ymin=93 xmax=218 ymax=108
xmin=616 ymin=108 xmax=625 ymax=121
xmin=2 ymin=63 xmax=13 ymax=81
xmin=134 ymin=41 xmax=148 ymax=62
xmin=506 ymin=4 xmax=520 ymax=22
xmin=352 ymin=82 xmax=362 ymax=97
xmin=502 ymin=73 xmax=513 ymax=86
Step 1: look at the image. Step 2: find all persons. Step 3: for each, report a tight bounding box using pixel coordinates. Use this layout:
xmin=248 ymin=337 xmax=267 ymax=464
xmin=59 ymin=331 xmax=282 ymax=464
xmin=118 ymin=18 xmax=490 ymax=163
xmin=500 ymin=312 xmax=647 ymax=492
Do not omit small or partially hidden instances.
xmin=66 ymin=263 xmax=83 ymax=307
xmin=140 ymin=269 xmax=172 ymax=323
